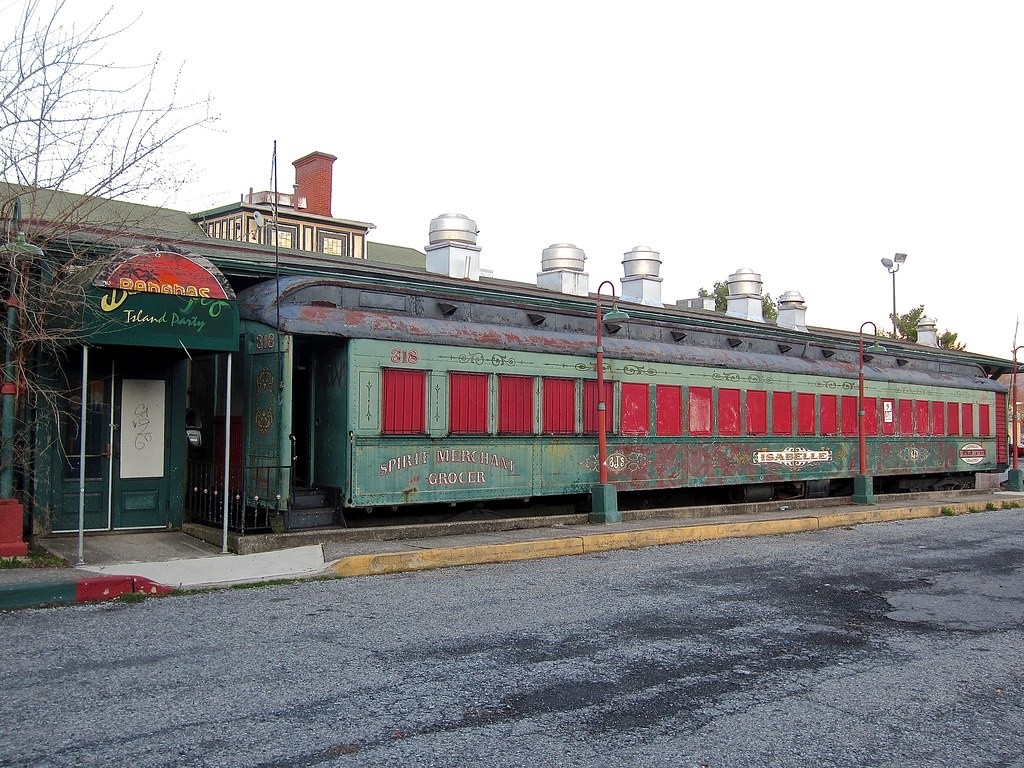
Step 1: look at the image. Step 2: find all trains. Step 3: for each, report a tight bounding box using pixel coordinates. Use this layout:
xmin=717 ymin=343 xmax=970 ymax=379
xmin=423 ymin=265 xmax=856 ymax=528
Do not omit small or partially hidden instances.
xmin=190 ymin=272 xmax=1009 ymax=532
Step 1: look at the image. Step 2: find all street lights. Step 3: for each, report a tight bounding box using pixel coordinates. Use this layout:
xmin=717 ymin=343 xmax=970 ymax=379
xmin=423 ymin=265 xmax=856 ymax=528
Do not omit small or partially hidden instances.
xmin=881 ymin=255 xmax=908 ymax=339
xmin=589 ymin=282 xmax=630 ymax=524
xmin=850 ymin=321 xmax=889 ymax=505
